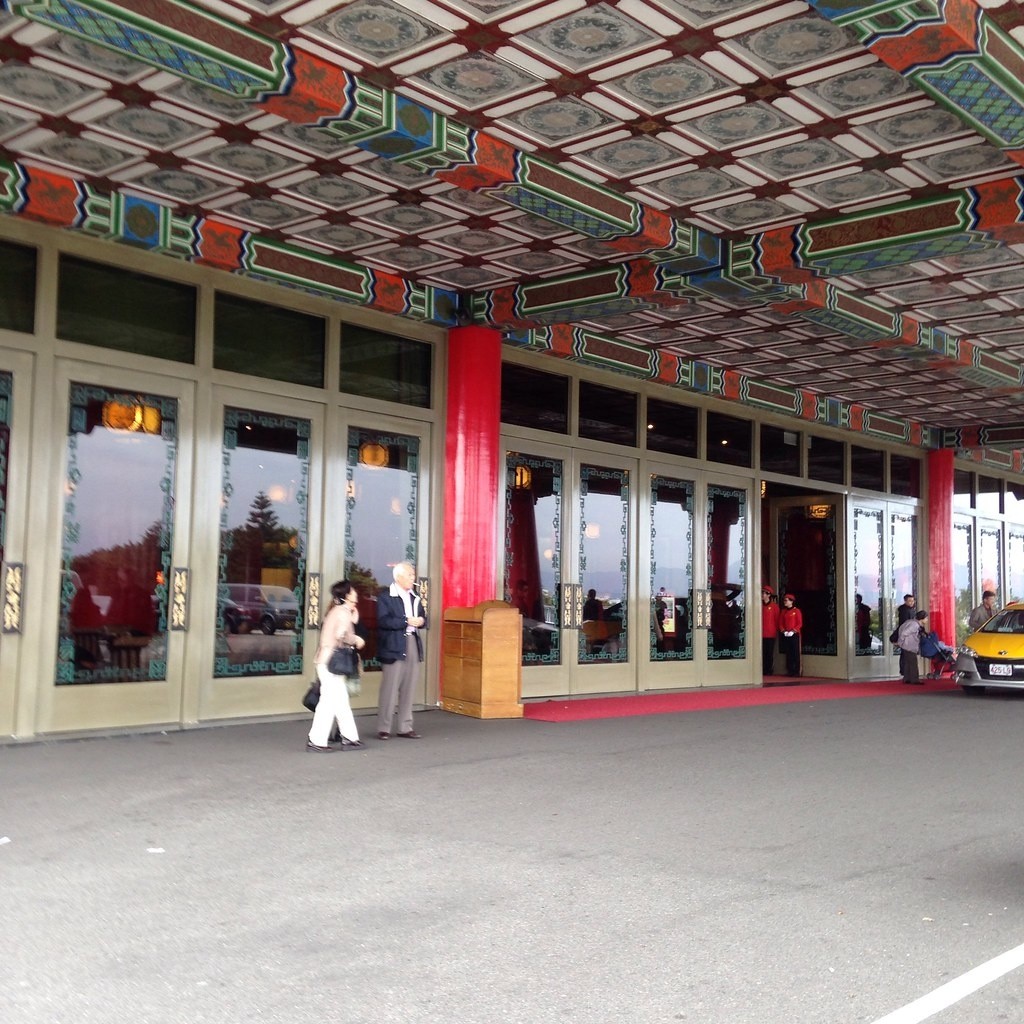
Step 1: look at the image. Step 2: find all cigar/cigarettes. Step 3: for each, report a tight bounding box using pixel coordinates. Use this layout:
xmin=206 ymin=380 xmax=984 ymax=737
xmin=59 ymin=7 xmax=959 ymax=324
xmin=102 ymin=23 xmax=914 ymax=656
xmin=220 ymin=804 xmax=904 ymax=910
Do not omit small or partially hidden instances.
xmin=413 ymin=583 xmax=420 ymax=586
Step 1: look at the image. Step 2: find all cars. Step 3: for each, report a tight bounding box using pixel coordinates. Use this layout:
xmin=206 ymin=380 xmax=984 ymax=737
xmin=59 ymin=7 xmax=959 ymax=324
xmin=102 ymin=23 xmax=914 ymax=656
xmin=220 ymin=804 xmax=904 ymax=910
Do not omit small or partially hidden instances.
xmin=949 ymin=601 xmax=1024 ymax=693
xmin=216 ymin=595 xmax=253 ymax=634
xmin=584 ymin=600 xmax=626 ymax=642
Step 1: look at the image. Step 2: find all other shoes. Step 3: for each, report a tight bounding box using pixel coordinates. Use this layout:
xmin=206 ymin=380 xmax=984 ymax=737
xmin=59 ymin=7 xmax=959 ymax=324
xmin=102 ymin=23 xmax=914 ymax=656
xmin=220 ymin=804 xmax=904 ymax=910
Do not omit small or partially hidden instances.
xmin=398 ymin=730 xmax=421 ymax=739
xmin=306 ymin=741 xmax=333 ymax=752
xmin=342 ymin=740 xmax=366 ymax=751
xmin=912 ymin=682 xmax=925 ymax=685
xmin=379 ymin=731 xmax=389 ymax=740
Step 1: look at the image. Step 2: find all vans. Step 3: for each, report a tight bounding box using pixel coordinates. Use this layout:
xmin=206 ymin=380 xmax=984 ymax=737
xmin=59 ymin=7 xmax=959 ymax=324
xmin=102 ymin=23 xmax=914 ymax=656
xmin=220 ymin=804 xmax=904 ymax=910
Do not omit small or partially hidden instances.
xmin=216 ymin=581 xmax=301 ymax=637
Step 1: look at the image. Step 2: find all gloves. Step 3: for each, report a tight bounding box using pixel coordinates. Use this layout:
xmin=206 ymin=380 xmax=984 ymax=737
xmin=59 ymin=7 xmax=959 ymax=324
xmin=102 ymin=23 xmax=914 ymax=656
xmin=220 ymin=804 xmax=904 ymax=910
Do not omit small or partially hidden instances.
xmin=784 ymin=631 xmax=789 ymax=636
xmin=787 ymin=631 xmax=794 ymax=637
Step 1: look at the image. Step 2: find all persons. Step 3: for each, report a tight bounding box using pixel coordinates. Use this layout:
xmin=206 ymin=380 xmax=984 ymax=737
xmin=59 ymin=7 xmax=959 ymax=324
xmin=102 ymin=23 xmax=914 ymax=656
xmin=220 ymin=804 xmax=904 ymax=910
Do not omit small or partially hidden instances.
xmin=762 ymin=585 xmax=802 ymax=677
xmin=855 ymin=594 xmax=872 ymax=650
xmin=969 ymin=591 xmax=1018 ymax=632
xmin=515 ymin=578 xmax=667 ymax=660
xmin=898 ymin=594 xmax=928 ymax=685
xmin=376 ymin=561 xmax=427 ymax=740
xmin=307 ymin=581 xmax=371 ymax=753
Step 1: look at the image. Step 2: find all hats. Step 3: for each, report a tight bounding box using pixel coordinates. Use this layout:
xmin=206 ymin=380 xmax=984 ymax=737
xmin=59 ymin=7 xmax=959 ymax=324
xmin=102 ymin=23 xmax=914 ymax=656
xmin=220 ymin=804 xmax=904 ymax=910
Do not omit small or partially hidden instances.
xmin=784 ymin=594 xmax=796 ymax=602
xmin=762 ymin=586 xmax=775 ymax=594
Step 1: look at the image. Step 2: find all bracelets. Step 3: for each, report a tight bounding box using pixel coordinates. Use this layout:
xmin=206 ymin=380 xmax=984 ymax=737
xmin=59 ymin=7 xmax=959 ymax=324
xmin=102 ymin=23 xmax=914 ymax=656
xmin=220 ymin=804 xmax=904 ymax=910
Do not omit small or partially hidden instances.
xmin=406 ymin=617 xmax=408 ymax=624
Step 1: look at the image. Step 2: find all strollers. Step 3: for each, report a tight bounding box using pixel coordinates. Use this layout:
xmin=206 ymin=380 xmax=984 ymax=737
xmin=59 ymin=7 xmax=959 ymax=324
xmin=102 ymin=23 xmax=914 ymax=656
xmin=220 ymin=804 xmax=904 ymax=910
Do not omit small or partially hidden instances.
xmin=918 ymin=627 xmax=959 ymax=681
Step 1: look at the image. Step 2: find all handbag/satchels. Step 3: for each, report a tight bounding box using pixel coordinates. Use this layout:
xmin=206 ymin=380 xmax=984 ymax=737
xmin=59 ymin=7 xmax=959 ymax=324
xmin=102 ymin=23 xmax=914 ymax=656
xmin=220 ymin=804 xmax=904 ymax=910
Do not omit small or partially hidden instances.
xmin=890 ymin=626 xmax=899 ymax=643
xmin=304 ymin=681 xmax=322 ymax=712
xmin=329 ymin=646 xmax=358 ymax=676
